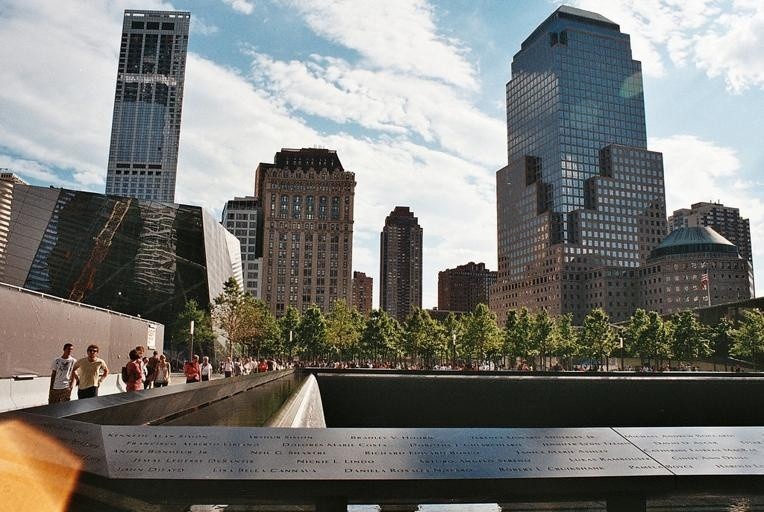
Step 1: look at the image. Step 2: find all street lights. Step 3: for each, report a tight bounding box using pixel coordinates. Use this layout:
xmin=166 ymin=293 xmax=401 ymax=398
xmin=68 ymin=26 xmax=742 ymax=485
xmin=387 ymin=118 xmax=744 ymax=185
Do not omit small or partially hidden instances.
xmin=188 ymin=319 xmax=195 ymax=362
xmin=618 ymin=336 xmax=625 ymax=370
xmin=452 ymin=333 xmax=456 ymax=364
xmin=289 ymin=330 xmax=293 ymax=362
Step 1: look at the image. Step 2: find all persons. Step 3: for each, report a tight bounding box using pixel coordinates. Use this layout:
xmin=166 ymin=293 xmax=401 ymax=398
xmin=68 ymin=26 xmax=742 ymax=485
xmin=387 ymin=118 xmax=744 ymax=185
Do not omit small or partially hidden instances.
xmin=617 ymin=363 xmax=700 ymax=372
xmin=551 ymin=363 xmax=604 ymax=372
xmin=730 ymin=366 xmax=745 ymax=372
xmin=72 ymin=344 xmax=109 ymax=399
xmin=125 ymin=343 xmax=295 ymax=405
xmin=297 ymin=360 xmax=534 ymax=373
xmin=47 ymin=342 xmax=77 ymax=404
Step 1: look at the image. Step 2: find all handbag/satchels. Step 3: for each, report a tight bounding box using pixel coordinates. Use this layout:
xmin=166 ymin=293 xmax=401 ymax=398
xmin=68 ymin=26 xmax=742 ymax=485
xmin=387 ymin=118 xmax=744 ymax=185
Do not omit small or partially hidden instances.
xmin=122 ymin=362 xmax=130 ymax=384
